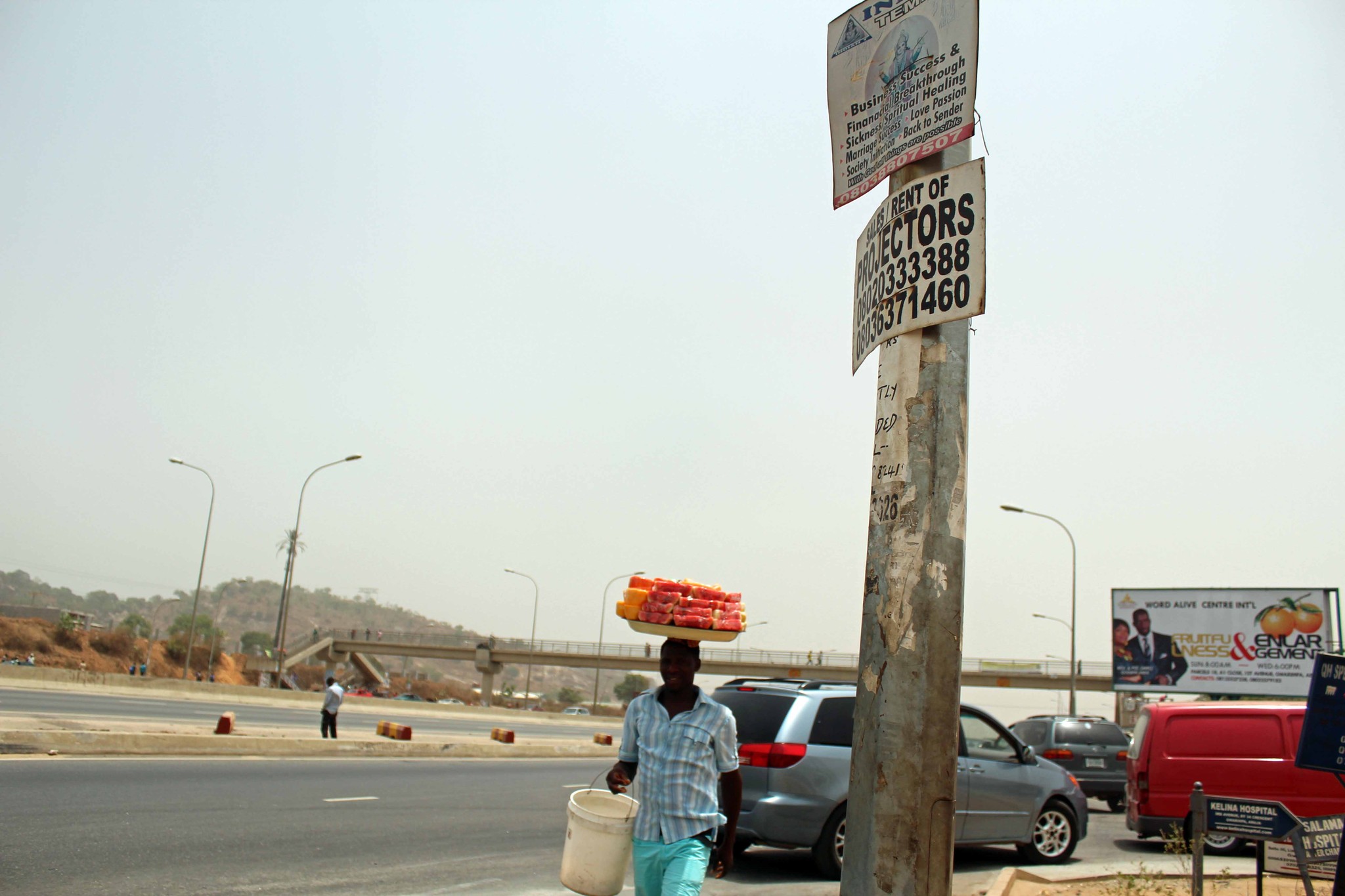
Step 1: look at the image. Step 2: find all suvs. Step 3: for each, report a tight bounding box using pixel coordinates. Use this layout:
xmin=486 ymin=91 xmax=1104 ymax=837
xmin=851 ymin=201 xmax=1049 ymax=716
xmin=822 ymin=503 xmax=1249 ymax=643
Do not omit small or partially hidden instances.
xmin=708 ymin=676 xmax=1090 ymax=882
xmin=978 ymin=712 xmax=1132 ymax=811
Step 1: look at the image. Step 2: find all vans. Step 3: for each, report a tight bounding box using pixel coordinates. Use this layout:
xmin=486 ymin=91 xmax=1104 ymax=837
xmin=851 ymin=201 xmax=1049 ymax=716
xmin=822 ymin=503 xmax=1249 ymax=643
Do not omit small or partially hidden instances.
xmin=1123 ymin=700 xmax=1345 ymax=859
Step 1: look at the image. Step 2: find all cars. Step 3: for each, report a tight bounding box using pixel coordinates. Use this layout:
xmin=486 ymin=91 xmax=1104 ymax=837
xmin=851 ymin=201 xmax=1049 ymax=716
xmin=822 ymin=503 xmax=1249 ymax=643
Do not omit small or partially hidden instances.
xmin=561 ymin=707 xmax=591 ymax=716
xmin=392 ymin=692 xmax=423 ymax=702
xmin=343 ymin=689 xmax=373 ymax=697
xmin=437 ymin=699 xmax=465 ymax=706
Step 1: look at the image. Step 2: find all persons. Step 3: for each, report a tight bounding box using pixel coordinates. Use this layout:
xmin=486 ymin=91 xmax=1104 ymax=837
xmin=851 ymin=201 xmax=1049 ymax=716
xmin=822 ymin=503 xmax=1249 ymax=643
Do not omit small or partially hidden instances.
xmin=1113 ymin=609 xmax=1188 ymax=685
xmin=490 ymin=636 xmax=496 ymax=650
xmin=406 ymin=682 xmax=412 ymax=693
xmin=347 ymin=684 xmax=350 ymax=691
xmin=130 ymin=661 xmax=147 ymax=676
xmin=606 ymin=638 xmax=743 ymax=896
xmin=350 ymin=628 xmax=383 ymax=641
xmin=644 ymin=643 xmax=651 ymax=657
xmin=79 ymin=660 xmax=86 ymax=671
xmin=1 ymin=653 xmax=35 ymax=666
xmin=1078 ymin=660 xmax=1081 ymax=675
xmin=288 ymin=669 xmax=296 ymax=683
xmin=805 ymin=651 xmax=823 ymax=666
xmin=321 ymin=677 xmax=343 ymax=738
xmin=313 ymin=629 xmax=317 ymax=643
xmin=195 ymin=670 xmax=214 ymax=682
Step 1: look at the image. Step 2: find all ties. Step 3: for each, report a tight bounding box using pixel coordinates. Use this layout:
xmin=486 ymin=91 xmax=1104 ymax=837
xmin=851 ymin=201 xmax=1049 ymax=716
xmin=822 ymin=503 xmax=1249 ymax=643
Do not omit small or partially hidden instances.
xmin=1143 ymin=638 xmax=1152 ymax=662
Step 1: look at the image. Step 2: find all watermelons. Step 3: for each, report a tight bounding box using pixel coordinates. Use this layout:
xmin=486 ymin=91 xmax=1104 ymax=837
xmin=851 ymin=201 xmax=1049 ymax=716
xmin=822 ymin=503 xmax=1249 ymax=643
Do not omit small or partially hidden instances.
xmin=629 ymin=576 xmax=742 ymax=631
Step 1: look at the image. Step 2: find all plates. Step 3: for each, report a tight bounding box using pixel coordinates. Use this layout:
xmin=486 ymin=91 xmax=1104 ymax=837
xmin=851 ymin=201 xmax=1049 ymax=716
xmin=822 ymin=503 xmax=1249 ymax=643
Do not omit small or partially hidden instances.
xmin=622 ymin=618 xmax=739 ymax=642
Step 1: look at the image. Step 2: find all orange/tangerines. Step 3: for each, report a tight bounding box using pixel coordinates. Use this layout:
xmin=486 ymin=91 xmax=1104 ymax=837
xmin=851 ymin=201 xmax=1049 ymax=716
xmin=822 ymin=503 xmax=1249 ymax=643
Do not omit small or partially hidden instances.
xmin=1261 ymin=603 xmax=1323 ymax=638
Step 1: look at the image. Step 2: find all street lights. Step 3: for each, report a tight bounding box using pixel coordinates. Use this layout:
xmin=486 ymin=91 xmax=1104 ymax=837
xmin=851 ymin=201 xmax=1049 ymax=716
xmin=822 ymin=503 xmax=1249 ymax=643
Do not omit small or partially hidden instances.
xmin=500 ymin=568 xmax=538 ymax=708
xmin=146 ymin=598 xmax=181 ymax=669
xmin=207 ymin=578 xmax=249 ymax=674
xmin=591 ymin=570 xmax=645 ymax=714
xmin=734 ymin=620 xmax=768 ymax=680
xmin=998 ymin=502 xmax=1078 ymax=716
xmin=167 ymin=455 xmax=216 ymax=678
xmin=276 ymin=453 xmax=367 ymax=692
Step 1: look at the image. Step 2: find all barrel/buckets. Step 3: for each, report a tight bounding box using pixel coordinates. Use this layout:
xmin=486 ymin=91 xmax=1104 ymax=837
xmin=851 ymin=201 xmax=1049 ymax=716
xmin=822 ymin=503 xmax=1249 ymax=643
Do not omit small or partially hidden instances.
xmin=559 ymin=767 xmax=638 ymax=896
